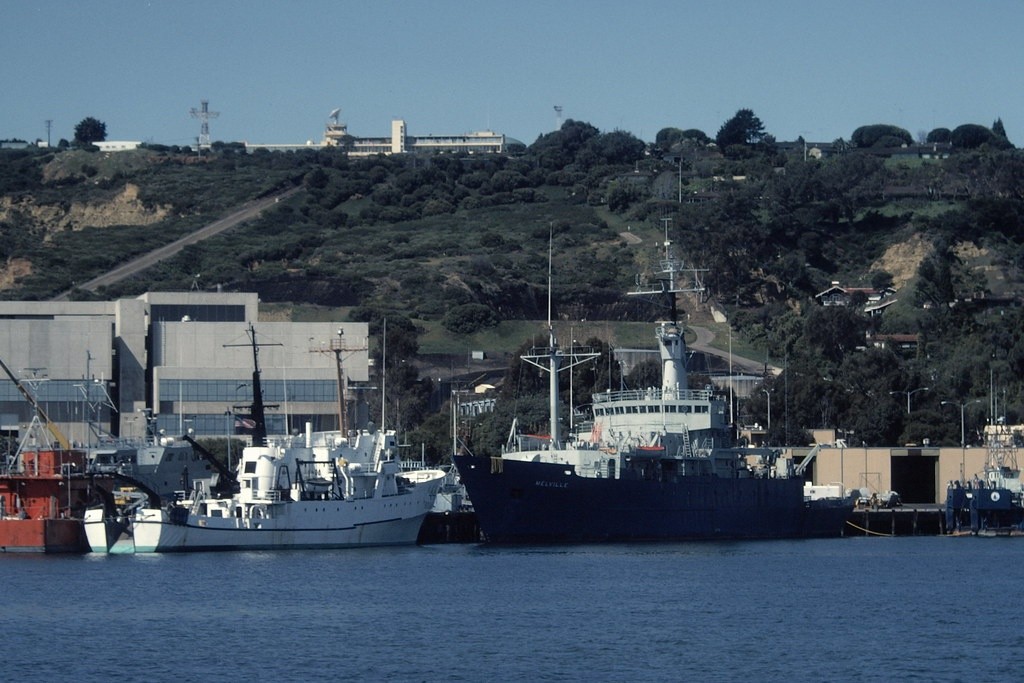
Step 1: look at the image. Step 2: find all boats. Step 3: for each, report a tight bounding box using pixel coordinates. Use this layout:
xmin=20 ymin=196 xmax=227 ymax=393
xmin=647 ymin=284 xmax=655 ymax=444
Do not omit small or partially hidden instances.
xmin=0 ymin=367 xmax=92 ymax=554
xmin=83 ymin=320 xmax=446 ymax=556
xmin=453 ymin=217 xmax=807 ymax=546
xmin=944 ymin=368 xmax=1024 ymax=539
xmin=514 ymin=433 xmax=550 ymax=451
xmin=633 ymin=445 xmax=665 ymax=459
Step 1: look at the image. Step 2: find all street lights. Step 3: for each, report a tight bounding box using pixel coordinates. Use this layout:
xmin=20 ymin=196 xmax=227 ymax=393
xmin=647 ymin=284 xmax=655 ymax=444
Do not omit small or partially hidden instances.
xmin=941 ymin=399 xmax=982 ymax=485
xmin=890 ymin=387 xmax=929 ymax=414
